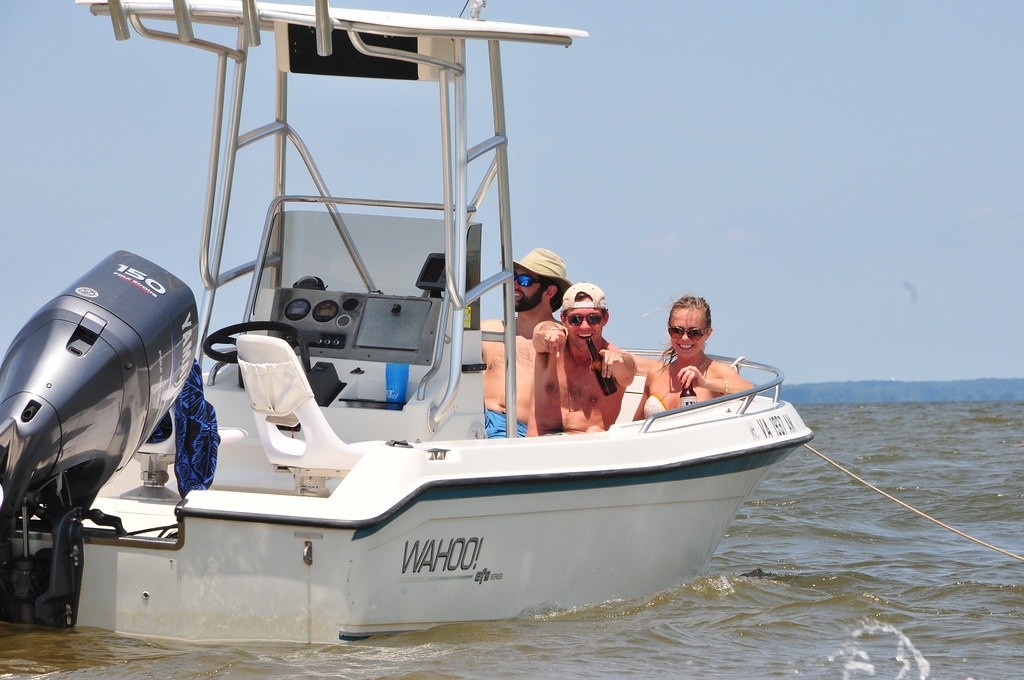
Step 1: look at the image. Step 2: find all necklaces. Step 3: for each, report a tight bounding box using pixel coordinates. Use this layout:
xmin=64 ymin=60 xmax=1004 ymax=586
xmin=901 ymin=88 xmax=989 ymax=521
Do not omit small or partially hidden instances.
xmin=699 ymin=360 xmax=706 ymax=369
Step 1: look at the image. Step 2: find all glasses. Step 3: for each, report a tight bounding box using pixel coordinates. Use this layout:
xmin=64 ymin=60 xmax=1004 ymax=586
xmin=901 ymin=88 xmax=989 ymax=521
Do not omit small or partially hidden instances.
xmin=668 ymin=326 xmax=708 ymax=340
xmin=564 ymin=315 xmax=603 ymax=326
xmin=514 ymin=271 xmax=541 ymax=287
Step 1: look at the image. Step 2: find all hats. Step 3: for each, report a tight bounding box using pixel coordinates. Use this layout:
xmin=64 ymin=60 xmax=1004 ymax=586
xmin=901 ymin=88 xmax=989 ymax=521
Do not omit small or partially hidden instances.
xmin=513 ymin=247 xmax=573 ymax=295
xmin=562 ymin=283 xmax=607 ymax=312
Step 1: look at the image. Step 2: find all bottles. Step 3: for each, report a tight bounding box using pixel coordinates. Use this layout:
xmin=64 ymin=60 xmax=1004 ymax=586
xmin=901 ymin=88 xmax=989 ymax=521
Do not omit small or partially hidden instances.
xmin=681 ymin=383 xmax=697 ymax=410
xmin=584 ymin=338 xmax=618 ymax=396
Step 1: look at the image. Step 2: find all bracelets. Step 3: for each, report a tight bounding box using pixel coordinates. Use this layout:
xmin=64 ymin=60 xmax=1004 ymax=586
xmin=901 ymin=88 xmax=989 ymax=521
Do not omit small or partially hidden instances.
xmin=720 ymin=378 xmax=729 ymax=395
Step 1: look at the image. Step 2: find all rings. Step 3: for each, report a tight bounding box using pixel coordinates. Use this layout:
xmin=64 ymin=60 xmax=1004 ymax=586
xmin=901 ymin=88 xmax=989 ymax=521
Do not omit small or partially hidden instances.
xmin=545 ymin=335 xmax=551 ymax=337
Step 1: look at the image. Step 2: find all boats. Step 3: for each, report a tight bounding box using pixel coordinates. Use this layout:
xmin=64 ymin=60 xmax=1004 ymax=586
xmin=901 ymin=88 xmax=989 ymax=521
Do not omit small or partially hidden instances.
xmin=2 ymin=1 xmax=817 ymax=646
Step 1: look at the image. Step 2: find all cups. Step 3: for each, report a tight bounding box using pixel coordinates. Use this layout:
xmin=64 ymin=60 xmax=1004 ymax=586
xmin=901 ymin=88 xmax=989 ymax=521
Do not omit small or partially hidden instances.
xmin=384 ymin=362 xmax=411 ymax=409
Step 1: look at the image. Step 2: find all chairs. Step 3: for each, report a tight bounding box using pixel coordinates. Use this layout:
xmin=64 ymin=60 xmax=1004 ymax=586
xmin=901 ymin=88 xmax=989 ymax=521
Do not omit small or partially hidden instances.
xmin=119 ymin=399 xmax=249 ymax=502
xmin=235 ymin=334 xmax=388 ymax=497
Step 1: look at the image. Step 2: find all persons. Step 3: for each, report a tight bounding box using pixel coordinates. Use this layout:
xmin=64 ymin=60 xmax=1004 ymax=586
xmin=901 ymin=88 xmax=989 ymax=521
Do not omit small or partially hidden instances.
xmin=526 ymin=282 xmax=637 ymax=438
xmin=481 ymin=248 xmax=573 ymax=439
xmin=632 ymin=296 xmax=754 ymax=421
